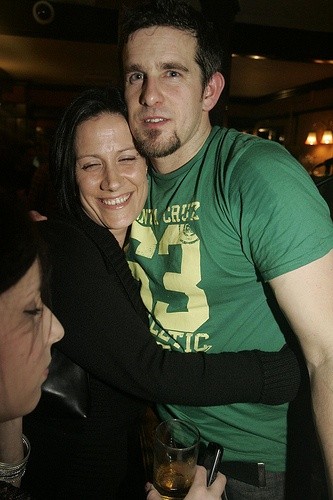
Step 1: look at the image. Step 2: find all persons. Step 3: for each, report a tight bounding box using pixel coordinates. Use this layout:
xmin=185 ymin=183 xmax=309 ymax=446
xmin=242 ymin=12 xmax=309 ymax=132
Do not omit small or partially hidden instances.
xmin=0 ymin=221 xmax=227 ymax=500
xmin=0 ymin=101 xmax=55 ymax=214
xmin=26 ymin=0 xmax=333 ymax=500
xmin=27 ymin=88 xmax=308 ymax=500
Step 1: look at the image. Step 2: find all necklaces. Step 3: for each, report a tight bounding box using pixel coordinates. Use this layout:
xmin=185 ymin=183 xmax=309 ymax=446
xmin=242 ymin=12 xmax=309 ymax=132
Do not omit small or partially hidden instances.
xmin=0 ymin=434 xmax=32 ymax=482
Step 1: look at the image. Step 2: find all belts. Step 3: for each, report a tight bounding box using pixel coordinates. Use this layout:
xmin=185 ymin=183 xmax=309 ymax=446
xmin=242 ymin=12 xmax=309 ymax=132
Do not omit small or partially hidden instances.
xmin=218 ymin=459 xmax=266 ymax=488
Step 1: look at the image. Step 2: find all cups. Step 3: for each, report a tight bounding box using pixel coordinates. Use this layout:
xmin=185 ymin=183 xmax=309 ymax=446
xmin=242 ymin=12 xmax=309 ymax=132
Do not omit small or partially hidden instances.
xmin=153 ymin=418 xmax=200 ymax=500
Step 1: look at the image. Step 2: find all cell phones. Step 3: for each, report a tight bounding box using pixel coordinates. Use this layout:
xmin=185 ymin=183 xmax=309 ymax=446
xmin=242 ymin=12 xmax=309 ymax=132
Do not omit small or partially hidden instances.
xmin=202 ymin=442 xmax=224 ymax=488
